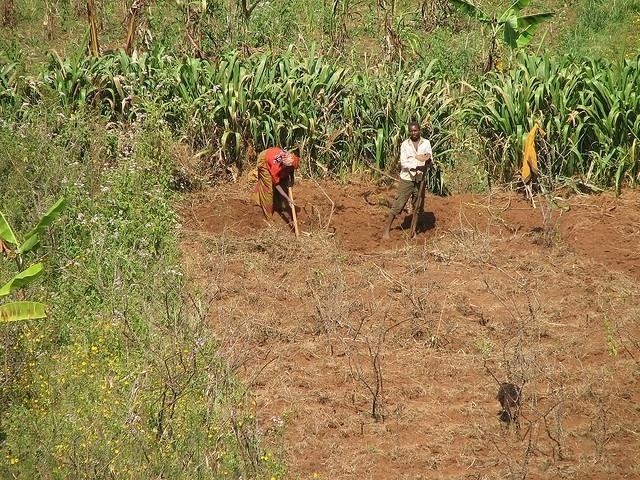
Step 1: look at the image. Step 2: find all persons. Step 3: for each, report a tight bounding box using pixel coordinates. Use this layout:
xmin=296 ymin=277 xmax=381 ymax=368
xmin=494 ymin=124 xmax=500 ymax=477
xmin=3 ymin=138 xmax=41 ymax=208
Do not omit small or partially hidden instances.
xmin=381 ymin=121 xmax=436 ymax=242
xmin=252 ymin=146 xmax=299 ymax=220
